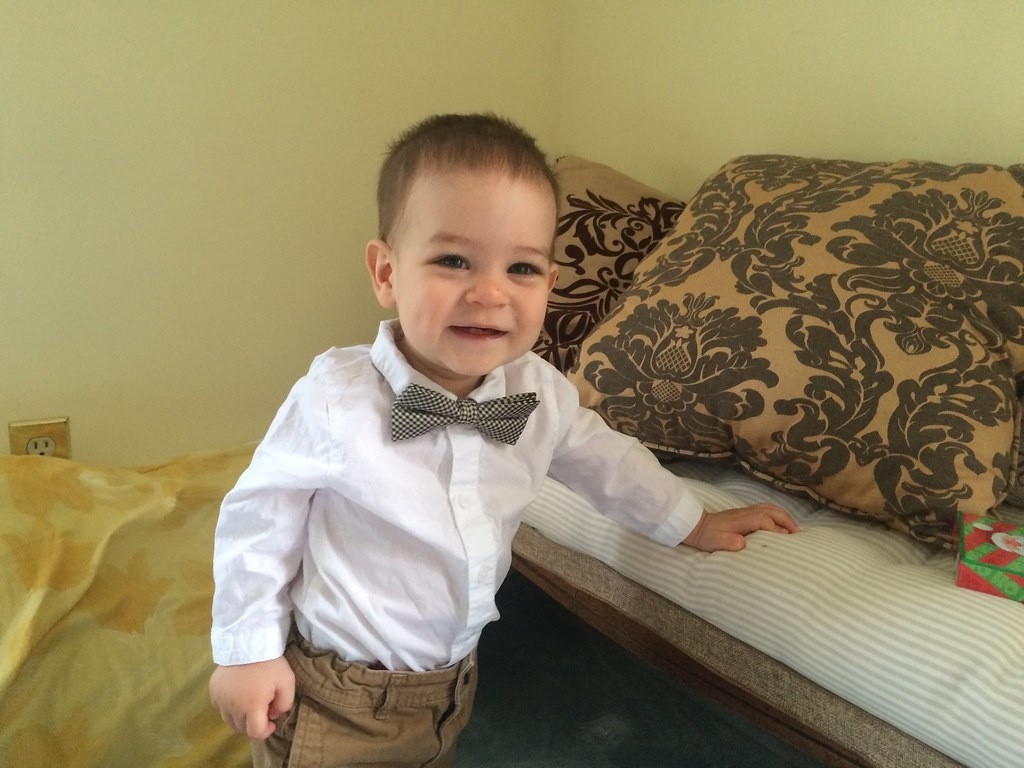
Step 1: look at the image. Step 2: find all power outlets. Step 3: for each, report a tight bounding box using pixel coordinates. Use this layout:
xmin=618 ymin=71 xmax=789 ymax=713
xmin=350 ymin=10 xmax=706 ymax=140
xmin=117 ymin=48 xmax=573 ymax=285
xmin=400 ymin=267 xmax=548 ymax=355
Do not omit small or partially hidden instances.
xmin=9 ymin=416 xmax=72 ymax=462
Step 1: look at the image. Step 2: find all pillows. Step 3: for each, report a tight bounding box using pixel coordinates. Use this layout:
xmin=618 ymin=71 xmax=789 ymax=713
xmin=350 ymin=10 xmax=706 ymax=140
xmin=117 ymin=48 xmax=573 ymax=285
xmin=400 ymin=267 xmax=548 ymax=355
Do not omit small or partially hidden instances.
xmin=566 ymin=155 xmax=1024 ymax=556
xmin=530 ymin=148 xmax=686 ymax=379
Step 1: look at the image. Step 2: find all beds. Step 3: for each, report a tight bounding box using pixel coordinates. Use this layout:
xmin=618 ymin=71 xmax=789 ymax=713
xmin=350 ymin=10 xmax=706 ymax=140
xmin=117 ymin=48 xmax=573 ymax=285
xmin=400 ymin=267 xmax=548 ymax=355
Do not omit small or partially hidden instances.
xmin=0 ymin=435 xmax=263 ymax=768
xmin=506 ymin=454 xmax=1024 ymax=768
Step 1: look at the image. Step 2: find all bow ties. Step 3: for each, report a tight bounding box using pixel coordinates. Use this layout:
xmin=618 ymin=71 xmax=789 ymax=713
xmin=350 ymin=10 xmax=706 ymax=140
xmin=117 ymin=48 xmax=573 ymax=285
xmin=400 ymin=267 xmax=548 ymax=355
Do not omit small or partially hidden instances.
xmin=391 ymin=383 xmax=540 ymax=446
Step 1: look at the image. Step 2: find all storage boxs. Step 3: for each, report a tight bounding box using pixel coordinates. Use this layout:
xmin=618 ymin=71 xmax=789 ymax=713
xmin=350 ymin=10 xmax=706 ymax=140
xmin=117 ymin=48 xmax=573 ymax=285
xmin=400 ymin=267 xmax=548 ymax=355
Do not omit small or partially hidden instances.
xmin=952 ymin=511 xmax=1024 ymax=605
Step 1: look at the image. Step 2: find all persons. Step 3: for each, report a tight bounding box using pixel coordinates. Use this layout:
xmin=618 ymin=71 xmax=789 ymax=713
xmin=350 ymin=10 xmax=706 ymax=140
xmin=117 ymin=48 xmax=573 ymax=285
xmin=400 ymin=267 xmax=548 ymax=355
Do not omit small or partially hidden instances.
xmin=209 ymin=113 xmax=802 ymax=768
